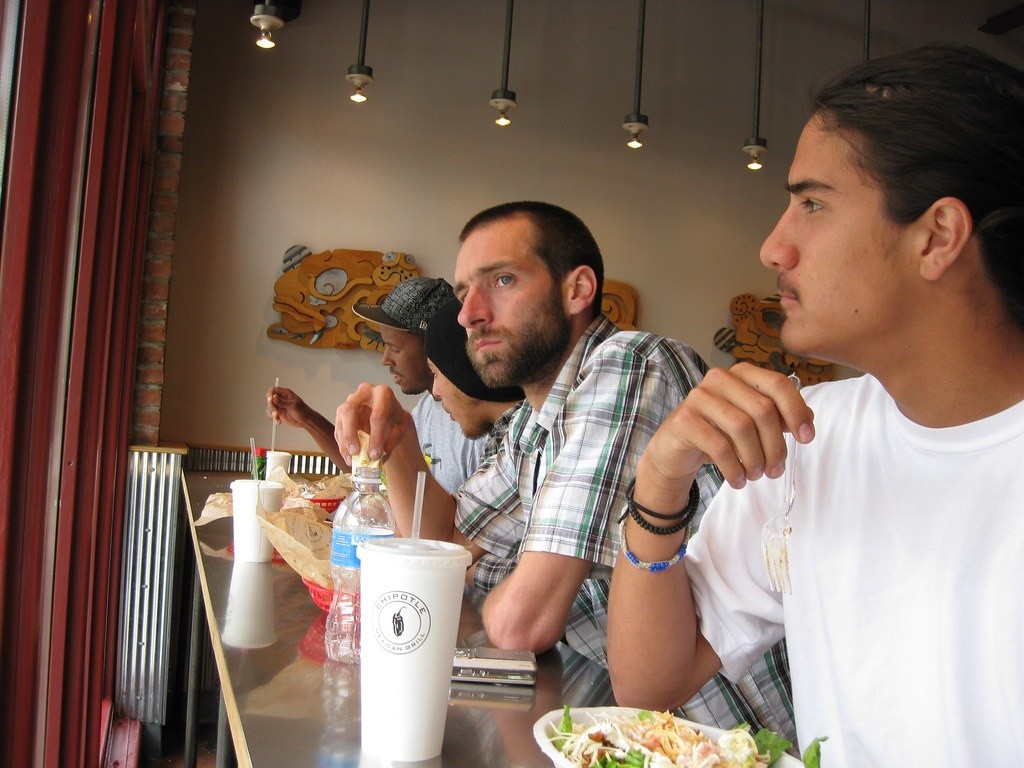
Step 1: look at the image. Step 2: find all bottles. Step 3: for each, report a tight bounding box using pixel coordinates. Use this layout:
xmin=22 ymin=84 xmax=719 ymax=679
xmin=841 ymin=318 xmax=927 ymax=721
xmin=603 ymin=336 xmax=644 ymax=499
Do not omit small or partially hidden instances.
xmin=251 ymin=448 xmax=266 ymax=480
xmin=324 ymin=467 xmax=394 ymax=663
xmin=316 ymin=657 xmax=361 ymax=767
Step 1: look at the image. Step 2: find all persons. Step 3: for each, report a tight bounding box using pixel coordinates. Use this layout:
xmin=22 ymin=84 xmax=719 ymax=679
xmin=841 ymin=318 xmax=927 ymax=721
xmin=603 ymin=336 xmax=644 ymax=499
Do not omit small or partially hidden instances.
xmin=423 ymin=299 xmax=531 ymax=596
xmin=444 ymin=596 xmax=607 ymax=768
xmin=609 ymin=48 xmax=1024 ymax=768
xmin=266 ymin=276 xmax=489 ymax=498
xmin=333 ymin=201 xmax=801 ymax=755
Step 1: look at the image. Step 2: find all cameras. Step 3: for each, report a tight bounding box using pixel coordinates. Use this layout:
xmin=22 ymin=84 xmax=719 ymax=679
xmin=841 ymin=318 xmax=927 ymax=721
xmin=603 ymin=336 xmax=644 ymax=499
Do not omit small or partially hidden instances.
xmin=450 ymin=646 xmax=538 ymax=685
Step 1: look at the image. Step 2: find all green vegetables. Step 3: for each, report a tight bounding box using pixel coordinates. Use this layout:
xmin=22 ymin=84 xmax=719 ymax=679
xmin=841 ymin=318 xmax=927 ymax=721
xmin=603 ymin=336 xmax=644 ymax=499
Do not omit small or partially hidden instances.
xmin=552 ymin=705 xmax=827 ymax=768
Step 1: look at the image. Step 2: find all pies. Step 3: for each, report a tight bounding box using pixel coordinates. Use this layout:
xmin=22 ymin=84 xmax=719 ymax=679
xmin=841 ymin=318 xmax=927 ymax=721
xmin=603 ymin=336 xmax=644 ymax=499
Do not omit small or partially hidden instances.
xmin=351 ymin=429 xmax=387 ymax=478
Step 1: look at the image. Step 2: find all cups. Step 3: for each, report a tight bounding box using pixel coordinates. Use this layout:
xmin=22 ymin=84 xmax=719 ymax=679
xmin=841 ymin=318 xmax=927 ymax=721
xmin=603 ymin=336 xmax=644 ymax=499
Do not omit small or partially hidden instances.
xmin=266 ymin=451 xmax=292 ymax=481
xmin=231 ymin=478 xmax=285 ymax=563
xmin=218 ymin=559 xmax=281 ymax=652
xmin=356 ymin=538 xmax=472 ymax=762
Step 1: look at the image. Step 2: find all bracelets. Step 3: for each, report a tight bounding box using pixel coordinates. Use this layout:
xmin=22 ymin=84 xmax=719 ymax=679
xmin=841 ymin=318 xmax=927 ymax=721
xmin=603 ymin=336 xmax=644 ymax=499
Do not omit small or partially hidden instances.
xmin=617 ymin=506 xmax=692 ymax=573
xmin=625 ymin=476 xmax=700 ymax=535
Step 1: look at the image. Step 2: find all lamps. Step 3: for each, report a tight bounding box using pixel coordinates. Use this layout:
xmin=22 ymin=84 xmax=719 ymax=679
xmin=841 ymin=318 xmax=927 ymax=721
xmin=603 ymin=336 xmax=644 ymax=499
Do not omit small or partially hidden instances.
xmin=344 ymin=1 xmax=375 ymax=102
xmin=624 ymin=1 xmax=650 ymax=149
xmin=744 ymin=1 xmax=767 ymax=169
xmin=250 ymin=1 xmax=284 ymax=50
xmin=490 ymin=0 xmax=517 ymax=125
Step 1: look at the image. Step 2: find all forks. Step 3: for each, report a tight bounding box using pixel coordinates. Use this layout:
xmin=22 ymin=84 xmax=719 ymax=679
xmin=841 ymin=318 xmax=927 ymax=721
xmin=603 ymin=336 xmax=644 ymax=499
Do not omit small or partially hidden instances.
xmin=760 ymin=373 xmax=801 ymax=595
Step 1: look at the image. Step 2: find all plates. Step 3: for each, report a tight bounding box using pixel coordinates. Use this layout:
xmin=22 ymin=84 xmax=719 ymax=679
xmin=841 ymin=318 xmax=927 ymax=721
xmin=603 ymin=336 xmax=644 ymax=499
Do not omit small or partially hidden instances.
xmin=533 ymin=706 xmax=805 ymax=768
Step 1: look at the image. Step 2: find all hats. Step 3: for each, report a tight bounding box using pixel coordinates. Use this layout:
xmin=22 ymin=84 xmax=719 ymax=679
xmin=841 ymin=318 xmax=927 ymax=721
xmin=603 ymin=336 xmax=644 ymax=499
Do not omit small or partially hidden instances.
xmin=423 ymin=297 xmax=527 ymax=402
xmin=351 ymin=277 xmax=461 ymax=335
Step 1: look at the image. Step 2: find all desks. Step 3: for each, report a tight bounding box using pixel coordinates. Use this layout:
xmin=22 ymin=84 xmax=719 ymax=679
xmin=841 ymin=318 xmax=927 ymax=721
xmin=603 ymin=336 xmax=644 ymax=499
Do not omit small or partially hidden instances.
xmin=179 ymin=466 xmax=692 ymax=767
